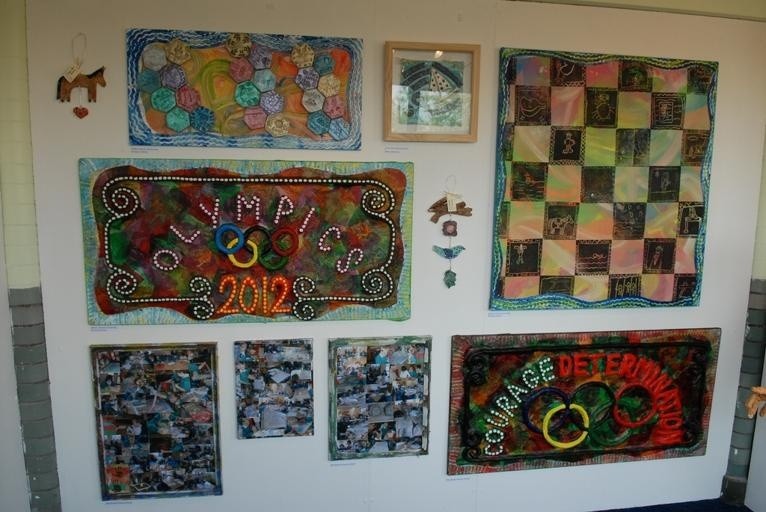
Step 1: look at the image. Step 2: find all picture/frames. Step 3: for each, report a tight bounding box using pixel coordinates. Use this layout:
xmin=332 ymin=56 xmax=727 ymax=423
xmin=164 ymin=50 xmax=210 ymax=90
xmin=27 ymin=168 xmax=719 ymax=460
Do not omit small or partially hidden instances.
xmin=384 ymin=40 xmax=481 ymax=143
